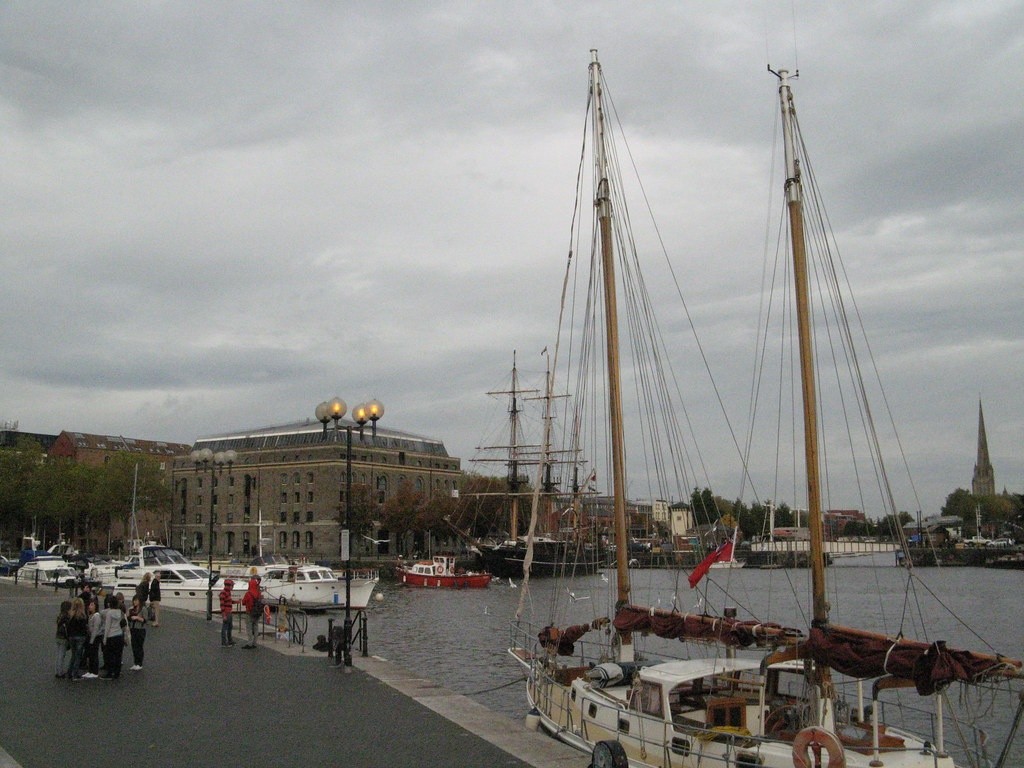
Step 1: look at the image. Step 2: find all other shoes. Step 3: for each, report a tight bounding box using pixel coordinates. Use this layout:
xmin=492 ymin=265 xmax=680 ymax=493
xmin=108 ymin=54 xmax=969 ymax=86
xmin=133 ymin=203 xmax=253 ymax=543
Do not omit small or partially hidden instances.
xmin=99 ymin=665 xmax=106 ymax=670
xmin=245 ymin=644 xmax=256 ymax=649
xmin=100 ymin=674 xmax=113 ymax=681
xmin=71 ymin=676 xmax=84 ymax=681
xmin=134 ymin=665 xmax=143 ymax=671
xmin=228 ymin=640 xmax=238 ymax=646
xmin=86 ymin=673 xmax=98 ymax=678
xmin=151 ymin=622 xmax=161 ymax=627
xmin=129 ymin=665 xmax=137 ymax=669
xmin=221 ymin=642 xmax=232 ymax=647
xmin=82 ymin=672 xmax=90 ymax=677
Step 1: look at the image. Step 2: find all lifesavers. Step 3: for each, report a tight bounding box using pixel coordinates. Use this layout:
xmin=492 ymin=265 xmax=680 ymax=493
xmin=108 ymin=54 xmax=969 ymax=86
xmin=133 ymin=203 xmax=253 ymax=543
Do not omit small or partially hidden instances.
xmin=437 ymin=566 xmax=444 ymax=573
xmin=450 ymin=566 xmax=455 ymax=573
xmin=792 ymin=725 xmax=846 ymax=767
xmin=250 ymin=566 xmax=257 ymax=575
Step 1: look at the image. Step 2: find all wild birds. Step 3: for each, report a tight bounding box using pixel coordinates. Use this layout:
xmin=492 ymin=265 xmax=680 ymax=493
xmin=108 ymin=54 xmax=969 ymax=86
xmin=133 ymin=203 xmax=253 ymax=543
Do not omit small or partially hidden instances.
xmin=360 ymin=517 xmax=720 ymax=616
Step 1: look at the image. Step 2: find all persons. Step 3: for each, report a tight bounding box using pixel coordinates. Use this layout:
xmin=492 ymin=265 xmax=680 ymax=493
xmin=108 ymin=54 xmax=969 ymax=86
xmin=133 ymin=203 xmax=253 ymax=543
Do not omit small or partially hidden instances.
xmin=219 ymin=579 xmax=241 ymax=648
xmin=136 ymin=572 xmax=161 ymax=627
xmin=242 ymin=575 xmax=261 ymax=648
xmin=55 ymin=583 xmax=149 ymax=683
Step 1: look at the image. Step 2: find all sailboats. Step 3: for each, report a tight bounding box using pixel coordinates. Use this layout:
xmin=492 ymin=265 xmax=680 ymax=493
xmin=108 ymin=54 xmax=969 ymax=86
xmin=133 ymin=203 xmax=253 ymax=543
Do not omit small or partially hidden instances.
xmin=434 ymin=345 xmax=615 ymax=578
xmin=506 ymin=43 xmax=1024 ymax=768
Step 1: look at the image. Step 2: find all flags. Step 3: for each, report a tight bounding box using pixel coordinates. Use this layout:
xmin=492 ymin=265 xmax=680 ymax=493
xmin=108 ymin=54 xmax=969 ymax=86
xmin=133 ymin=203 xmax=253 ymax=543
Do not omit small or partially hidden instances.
xmin=689 ymin=527 xmax=737 ymax=588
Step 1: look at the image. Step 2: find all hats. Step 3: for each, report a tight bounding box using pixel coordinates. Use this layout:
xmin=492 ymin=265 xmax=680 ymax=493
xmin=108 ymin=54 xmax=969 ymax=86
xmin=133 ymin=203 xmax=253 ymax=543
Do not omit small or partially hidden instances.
xmin=224 ymin=579 xmax=234 ymax=585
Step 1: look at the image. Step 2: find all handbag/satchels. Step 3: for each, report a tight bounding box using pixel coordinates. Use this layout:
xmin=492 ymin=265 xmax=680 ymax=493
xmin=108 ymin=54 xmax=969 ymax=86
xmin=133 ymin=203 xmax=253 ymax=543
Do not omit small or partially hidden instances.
xmin=120 ymin=610 xmax=127 ymax=632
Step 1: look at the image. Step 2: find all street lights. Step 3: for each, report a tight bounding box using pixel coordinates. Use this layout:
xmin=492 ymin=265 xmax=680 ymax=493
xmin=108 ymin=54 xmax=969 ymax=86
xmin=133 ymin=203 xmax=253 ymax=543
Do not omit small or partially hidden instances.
xmin=190 ymin=447 xmax=238 ymax=622
xmin=314 ymin=395 xmax=387 ymax=667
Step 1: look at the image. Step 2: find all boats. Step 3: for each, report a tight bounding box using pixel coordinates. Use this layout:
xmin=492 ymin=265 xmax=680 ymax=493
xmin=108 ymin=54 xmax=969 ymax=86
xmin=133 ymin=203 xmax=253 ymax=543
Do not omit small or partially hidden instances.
xmin=393 ymin=553 xmax=494 ymax=590
xmin=0 ymin=461 xmax=381 ymax=614
xmin=708 ymin=557 xmax=747 ymax=570
